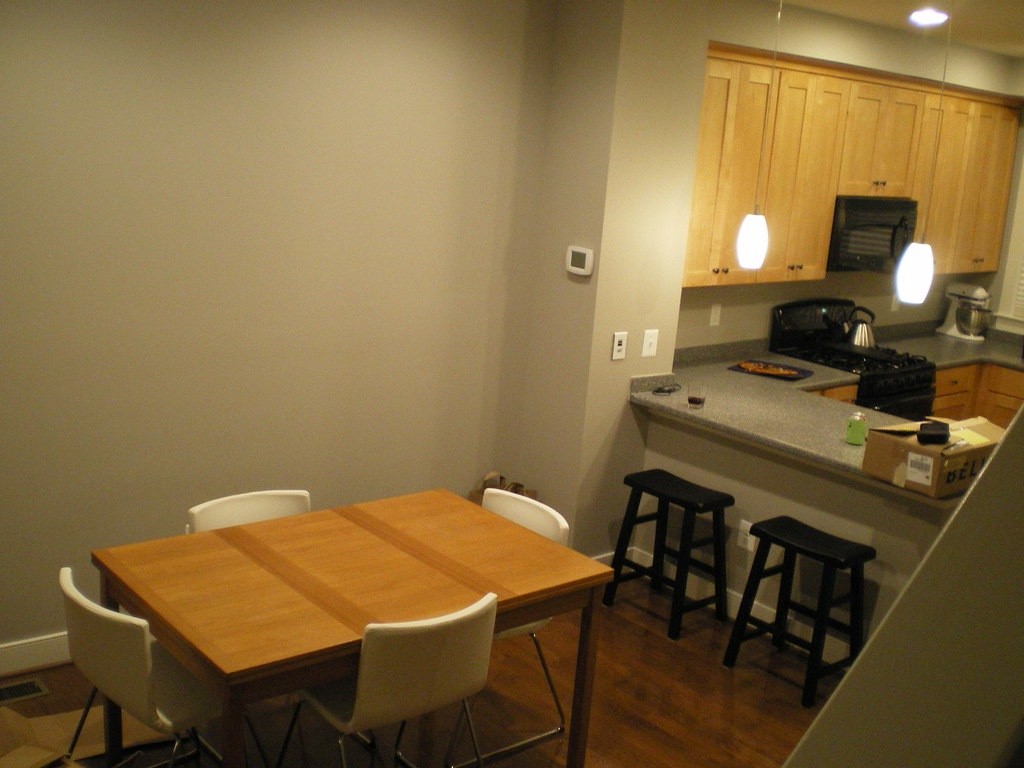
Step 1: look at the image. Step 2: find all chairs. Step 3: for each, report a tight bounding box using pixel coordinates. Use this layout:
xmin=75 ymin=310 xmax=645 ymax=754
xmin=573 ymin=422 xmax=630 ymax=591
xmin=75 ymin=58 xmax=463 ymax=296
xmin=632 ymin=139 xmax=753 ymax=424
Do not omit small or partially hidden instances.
xmin=52 ymin=564 xmax=272 ymax=768
xmin=453 ymin=482 xmax=582 ymax=762
xmin=280 ymin=582 xmax=501 ymax=768
xmin=224 ymin=483 xmax=323 ymax=768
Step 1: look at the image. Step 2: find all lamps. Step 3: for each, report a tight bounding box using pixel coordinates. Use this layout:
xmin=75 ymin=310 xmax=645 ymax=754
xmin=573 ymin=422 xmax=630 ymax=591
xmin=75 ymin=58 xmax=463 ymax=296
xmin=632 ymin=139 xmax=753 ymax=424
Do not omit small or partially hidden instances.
xmin=892 ymin=0 xmax=953 ymax=312
xmin=731 ymin=0 xmax=793 ymax=271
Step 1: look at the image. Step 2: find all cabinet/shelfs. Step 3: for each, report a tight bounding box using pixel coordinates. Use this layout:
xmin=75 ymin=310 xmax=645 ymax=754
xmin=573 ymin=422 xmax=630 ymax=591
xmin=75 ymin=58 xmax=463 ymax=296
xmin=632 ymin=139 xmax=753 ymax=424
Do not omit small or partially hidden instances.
xmin=684 ymin=40 xmax=1018 ymax=289
xmin=803 ymin=365 xmax=1024 ymax=429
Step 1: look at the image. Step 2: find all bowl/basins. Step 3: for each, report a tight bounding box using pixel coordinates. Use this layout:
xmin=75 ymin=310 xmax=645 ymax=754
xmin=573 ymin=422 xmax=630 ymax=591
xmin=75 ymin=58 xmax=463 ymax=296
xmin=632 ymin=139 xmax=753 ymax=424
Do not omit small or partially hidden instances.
xmin=959 ymin=307 xmax=991 ymax=336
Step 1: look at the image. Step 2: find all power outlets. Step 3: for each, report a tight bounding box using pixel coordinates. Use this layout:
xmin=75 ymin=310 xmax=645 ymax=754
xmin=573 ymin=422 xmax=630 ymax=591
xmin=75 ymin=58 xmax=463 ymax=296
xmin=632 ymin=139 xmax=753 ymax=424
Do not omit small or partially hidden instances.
xmin=737 ymin=520 xmax=757 ymax=551
xmin=710 ymin=303 xmax=722 ymax=327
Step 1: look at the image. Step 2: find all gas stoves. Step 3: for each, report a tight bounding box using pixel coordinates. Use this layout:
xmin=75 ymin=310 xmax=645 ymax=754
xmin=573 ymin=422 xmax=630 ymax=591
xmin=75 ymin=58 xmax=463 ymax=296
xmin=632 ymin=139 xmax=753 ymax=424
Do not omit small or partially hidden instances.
xmin=768 ymin=296 xmax=935 ymax=395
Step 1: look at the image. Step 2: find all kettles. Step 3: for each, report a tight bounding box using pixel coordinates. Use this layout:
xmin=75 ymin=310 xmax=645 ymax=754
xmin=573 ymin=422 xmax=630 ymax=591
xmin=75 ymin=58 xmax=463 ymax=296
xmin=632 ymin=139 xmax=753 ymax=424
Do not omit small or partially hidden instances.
xmin=841 ymin=305 xmax=876 ymax=348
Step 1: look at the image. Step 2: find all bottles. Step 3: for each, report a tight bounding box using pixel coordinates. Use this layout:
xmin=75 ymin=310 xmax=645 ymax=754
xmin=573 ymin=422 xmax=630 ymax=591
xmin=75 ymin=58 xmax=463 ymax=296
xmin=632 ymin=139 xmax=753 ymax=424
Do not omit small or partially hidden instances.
xmin=847 ymin=411 xmax=867 ymax=445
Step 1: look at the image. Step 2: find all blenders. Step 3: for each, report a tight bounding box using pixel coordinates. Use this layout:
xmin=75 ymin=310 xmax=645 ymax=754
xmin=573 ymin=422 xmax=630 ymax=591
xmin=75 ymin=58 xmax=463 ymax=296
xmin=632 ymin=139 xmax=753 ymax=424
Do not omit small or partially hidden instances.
xmin=934 ymin=281 xmax=990 ymax=341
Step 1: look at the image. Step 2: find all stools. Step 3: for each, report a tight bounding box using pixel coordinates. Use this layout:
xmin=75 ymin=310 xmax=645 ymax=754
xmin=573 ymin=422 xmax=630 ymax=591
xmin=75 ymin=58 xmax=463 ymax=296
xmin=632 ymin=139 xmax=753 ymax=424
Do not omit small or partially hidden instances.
xmin=722 ymin=512 xmax=884 ymax=701
xmin=602 ymin=456 xmax=736 ymax=646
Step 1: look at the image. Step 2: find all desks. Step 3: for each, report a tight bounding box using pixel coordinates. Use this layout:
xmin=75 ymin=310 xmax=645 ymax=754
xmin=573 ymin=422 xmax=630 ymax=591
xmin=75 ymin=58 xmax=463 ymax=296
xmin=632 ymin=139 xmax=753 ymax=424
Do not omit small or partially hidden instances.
xmin=90 ymin=481 xmax=623 ymax=768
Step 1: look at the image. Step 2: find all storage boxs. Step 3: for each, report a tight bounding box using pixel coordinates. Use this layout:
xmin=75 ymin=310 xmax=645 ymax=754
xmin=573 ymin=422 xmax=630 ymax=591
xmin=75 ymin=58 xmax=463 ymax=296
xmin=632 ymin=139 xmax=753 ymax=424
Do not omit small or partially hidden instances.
xmin=860 ymin=414 xmax=1006 ymax=499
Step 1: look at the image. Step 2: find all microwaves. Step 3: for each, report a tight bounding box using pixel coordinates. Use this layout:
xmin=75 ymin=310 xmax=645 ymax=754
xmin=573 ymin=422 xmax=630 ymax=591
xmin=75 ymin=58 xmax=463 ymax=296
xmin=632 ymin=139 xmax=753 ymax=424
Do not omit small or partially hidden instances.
xmin=827 ymin=195 xmax=918 ymax=271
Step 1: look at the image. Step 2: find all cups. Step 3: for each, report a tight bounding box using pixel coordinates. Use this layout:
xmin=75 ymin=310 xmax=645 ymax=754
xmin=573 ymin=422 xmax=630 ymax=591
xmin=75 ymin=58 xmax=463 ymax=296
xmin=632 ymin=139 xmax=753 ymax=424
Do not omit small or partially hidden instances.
xmin=688 ymin=383 xmax=707 ymax=408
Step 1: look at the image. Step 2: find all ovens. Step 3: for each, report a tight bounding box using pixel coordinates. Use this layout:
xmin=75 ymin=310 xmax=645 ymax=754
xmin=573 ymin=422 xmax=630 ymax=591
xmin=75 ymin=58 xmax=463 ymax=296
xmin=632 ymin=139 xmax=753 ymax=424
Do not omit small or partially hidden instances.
xmin=856 ymin=388 xmax=935 ymax=422
